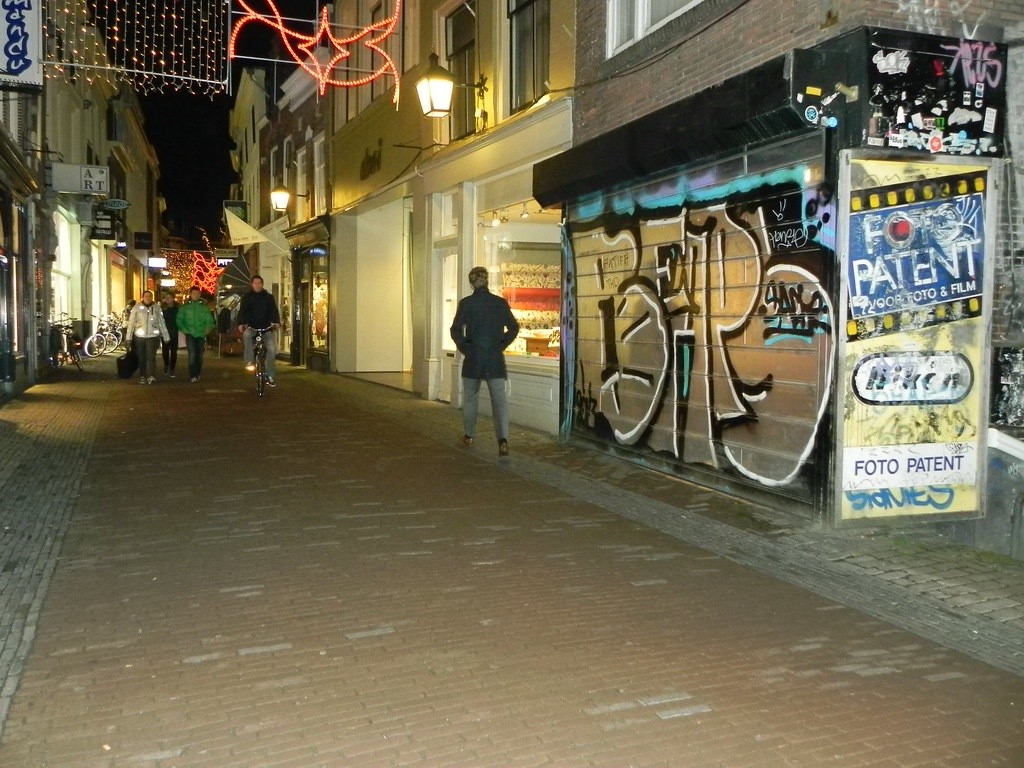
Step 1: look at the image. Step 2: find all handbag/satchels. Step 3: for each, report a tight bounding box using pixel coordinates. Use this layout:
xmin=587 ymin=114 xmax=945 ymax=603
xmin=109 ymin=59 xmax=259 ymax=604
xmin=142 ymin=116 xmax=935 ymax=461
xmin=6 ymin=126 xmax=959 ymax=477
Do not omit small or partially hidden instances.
xmin=116 ymin=347 xmax=139 ymax=378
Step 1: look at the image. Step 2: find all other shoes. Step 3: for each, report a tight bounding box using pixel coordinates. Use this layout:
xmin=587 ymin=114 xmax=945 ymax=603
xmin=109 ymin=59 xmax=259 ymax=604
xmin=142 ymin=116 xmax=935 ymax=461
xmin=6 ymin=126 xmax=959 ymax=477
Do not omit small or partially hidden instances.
xmin=191 ymin=376 xmax=197 ymax=383
xmin=498 ymin=442 xmax=510 ymax=456
xmin=164 ymin=367 xmax=169 ymax=376
xmin=169 ymin=369 xmax=175 ymax=378
xmin=461 ymin=434 xmax=474 ymax=446
xmin=265 ymin=376 xmax=277 ymax=387
xmin=147 ymin=376 xmax=157 ymax=385
xmin=139 ymin=376 xmax=147 ymax=384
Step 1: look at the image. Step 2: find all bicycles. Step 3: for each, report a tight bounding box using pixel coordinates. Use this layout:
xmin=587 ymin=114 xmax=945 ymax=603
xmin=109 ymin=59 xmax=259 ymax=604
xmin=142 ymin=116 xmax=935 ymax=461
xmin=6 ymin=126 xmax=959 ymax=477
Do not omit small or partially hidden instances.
xmin=240 ymin=323 xmax=279 ymax=397
xmin=50 ymin=312 xmax=127 ymax=371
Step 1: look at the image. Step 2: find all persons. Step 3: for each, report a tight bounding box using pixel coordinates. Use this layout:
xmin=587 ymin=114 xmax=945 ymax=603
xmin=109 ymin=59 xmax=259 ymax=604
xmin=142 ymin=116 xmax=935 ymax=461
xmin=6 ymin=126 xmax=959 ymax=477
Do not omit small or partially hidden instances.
xmin=159 ymin=293 xmax=181 ymax=379
xmin=176 ymin=285 xmax=215 ymax=384
xmin=126 ymin=300 xmax=136 ymax=321
xmin=237 ymin=275 xmax=280 ymax=388
xmin=450 ymin=267 xmax=519 ymax=457
xmin=124 ymin=291 xmax=170 ymax=384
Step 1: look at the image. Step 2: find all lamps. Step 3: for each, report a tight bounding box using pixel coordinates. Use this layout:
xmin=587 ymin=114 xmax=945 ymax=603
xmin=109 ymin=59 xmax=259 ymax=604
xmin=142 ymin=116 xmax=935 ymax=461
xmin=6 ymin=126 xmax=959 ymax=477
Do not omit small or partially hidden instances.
xmin=413 ymin=53 xmax=488 ymax=118
xmin=270 ymin=179 xmax=309 ymax=211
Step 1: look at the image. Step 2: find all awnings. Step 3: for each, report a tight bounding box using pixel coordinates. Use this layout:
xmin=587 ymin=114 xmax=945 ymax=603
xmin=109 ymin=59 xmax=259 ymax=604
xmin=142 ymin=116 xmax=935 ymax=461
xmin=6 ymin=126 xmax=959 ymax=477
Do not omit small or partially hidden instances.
xmin=218 ymin=253 xmax=250 ymax=293
